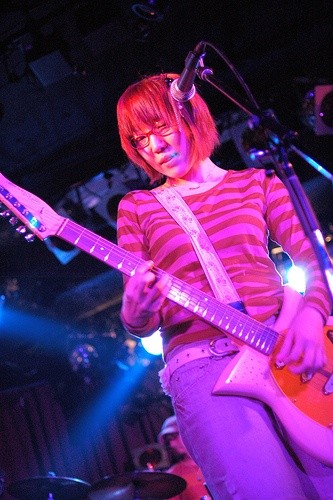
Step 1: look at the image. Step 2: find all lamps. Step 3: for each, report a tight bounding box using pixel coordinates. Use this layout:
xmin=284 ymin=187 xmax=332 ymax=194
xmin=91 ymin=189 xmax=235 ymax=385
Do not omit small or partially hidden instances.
xmin=41 ymin=85 xmax=333 ymax=251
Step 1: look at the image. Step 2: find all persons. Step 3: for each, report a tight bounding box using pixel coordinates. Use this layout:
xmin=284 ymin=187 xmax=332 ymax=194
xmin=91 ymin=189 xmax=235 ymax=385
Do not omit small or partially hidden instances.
xmin=158 ymin=416 xmax=212 ymax=500
xmin=117 ymin=73 xmax=333 ymax=500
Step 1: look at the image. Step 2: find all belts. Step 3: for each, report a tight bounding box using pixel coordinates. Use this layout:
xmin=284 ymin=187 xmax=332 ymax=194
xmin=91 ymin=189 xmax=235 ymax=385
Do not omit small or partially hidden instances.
xmin=160 ymin=334 xmax=240 ymax=375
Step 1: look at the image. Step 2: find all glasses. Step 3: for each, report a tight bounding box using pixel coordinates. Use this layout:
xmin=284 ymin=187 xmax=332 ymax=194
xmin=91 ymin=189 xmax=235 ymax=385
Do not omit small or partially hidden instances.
xmin=165 ymin=433 xmax=178 ymax=440
xmin=127 ymin=118 xmax=178 ymax=149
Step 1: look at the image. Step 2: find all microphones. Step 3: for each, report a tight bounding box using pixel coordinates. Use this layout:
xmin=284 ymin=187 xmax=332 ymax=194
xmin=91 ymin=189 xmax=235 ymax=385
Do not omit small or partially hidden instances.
xmin=170 ymin=41 xmax=206 ymax=102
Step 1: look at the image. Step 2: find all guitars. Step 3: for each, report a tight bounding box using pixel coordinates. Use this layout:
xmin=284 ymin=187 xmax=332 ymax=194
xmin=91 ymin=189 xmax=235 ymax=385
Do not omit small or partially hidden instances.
xmin=0 ymin=173 xmax=333 ymax=467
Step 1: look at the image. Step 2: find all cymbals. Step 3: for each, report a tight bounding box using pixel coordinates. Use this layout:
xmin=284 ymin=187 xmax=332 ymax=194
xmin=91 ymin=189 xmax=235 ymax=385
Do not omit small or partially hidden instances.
xmin=87 ymin=470 xmax=187 ymax=500
xmin=3 ymin=476 xmax=92 ymax=500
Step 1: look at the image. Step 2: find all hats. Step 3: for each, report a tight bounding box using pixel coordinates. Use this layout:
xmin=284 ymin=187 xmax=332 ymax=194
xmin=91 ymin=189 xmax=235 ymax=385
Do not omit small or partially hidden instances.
xmin=157 ymin=415 xmax=180 ymax=445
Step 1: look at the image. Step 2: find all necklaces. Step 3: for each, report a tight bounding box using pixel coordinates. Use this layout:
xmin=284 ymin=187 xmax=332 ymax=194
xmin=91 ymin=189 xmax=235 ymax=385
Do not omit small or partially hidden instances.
xmin=171 ymin=165 xmax=216 ymax=190
